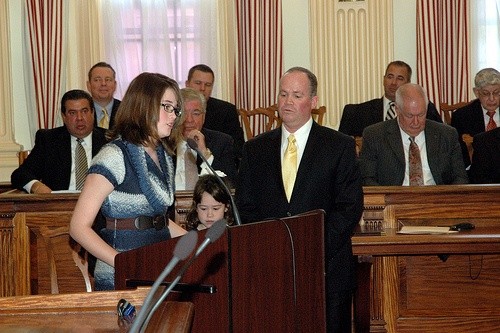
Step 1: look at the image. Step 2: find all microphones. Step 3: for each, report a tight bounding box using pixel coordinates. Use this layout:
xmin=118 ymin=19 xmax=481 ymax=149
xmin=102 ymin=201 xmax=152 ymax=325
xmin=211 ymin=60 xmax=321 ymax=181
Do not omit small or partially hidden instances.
xmin=187 ymin=138 xmax=241 ymax=224
xmin=140 ymin=218 xmax=228 ymax=333
xmin=129 ymin=230 xmax=198 ymax=332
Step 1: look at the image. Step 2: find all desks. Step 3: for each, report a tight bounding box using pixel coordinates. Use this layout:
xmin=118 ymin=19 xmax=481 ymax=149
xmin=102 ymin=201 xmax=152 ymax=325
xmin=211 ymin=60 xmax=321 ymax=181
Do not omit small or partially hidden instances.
xmin=2 ymin=181 xmax=500 ymax=333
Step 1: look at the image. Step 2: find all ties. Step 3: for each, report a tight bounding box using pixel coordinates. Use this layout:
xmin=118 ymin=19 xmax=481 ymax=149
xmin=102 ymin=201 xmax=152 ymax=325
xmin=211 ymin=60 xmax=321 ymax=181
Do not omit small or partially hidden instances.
xmin=76 ymin=138 xmax=89 ymax=191
xmin=184 ymin=143 xmax=200 ymax=191
xmin=282 ymin=135 xmax=297 ymax=203
xmin=385 ymin=101 xmax=396 ymax=121
xmin=408 ymin=137 xmax=424 ymax=185
xmin=99 ymin=108 xmax=110 ymax=130
xmin=486 ymin=110 xmax=497 ymax=130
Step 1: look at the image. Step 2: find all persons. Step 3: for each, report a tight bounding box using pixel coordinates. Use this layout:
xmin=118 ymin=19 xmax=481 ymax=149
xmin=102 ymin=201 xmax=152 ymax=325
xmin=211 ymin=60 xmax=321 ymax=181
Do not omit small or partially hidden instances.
xmin=352 ymin=59 xmax=442 ymax=132
xmin=183 ymin=64 xmax=245 ymax=148
xmin=185 ymin=174 xmax=236 ymax=233
xmin=234 ymin=67 xmax=364 ymax=332
xmin=357 ymin=83 xmax=469 ymax=189
xmin=470 ymin=124 xmax=500 ymax=184
xmin=449 ymin=68 xmax=500 ymax=160
xmin=86 ymin=61 xmax=125 ymax=132
xmin=67 ymin=73 xmax=189 ymax=292
xmin=174 ymin=87 xmax=240 ymax=192
xmin=10 ymin=89 xmax=111 ymax=194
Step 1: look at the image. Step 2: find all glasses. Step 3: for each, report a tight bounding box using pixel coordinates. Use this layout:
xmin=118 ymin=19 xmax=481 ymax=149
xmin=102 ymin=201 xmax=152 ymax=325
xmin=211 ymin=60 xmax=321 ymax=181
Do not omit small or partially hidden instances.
xmin=160 ymin=103 xmax=182 ymax=116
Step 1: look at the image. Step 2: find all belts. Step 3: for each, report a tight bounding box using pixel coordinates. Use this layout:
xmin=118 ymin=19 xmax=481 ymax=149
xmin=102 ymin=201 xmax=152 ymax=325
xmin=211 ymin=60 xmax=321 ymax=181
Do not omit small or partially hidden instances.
xmin=106 ymin=216 xmax=168 ymax=230
